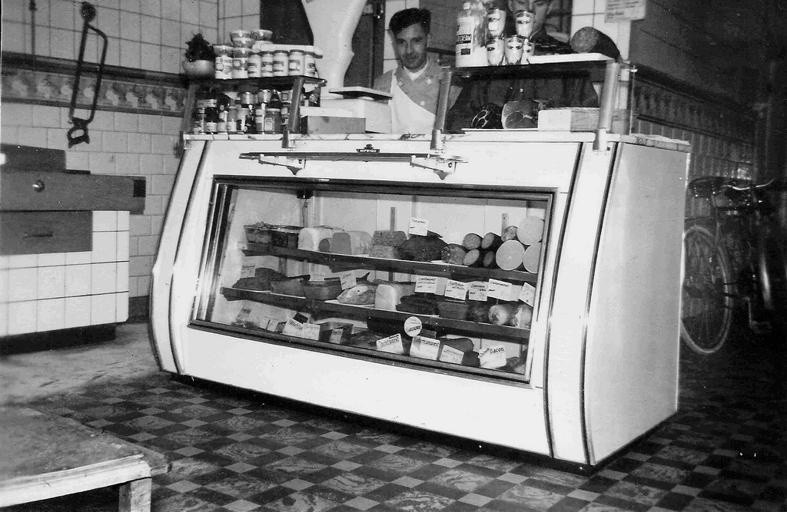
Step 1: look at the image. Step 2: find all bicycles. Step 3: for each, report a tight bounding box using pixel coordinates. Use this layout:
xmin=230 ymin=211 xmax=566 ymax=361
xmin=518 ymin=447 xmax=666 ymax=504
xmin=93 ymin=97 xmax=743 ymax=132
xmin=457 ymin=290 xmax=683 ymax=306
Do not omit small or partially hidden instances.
xmin=681 ymin=174 xmax=787 ymax=357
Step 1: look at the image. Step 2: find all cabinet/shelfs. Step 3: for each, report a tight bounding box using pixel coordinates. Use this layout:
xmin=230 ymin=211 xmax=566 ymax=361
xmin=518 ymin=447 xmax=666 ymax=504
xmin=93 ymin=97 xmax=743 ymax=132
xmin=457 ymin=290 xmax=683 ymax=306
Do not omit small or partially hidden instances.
xmin=147 ymin=127 xmax=692 ymax=476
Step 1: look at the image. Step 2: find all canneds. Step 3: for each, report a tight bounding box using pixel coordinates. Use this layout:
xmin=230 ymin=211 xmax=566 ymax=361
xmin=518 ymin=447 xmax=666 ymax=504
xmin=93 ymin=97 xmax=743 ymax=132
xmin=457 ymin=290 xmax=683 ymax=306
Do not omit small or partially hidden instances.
xmin=213 ymin=29 xmax=323 ymax=80
xmin=191 ymin=88 xmax=291 ymax=135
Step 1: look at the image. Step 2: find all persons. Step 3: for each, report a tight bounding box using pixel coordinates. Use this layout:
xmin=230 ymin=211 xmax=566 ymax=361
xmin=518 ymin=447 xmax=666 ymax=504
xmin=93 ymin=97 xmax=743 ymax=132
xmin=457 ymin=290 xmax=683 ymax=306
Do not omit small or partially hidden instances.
xmin=446 ymin=0 xmax=598 ymax=134
xmin=372 ymin=8 xmax=463 ymax=134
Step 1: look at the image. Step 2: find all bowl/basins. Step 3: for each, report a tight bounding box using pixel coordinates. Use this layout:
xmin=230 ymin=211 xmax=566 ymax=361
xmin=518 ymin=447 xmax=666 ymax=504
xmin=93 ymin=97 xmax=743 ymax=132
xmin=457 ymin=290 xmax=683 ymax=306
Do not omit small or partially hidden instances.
xmin=182 ymin=60 xmax=214 ymax=80
xmin=213 ymin=29 xmax=273 ymax=58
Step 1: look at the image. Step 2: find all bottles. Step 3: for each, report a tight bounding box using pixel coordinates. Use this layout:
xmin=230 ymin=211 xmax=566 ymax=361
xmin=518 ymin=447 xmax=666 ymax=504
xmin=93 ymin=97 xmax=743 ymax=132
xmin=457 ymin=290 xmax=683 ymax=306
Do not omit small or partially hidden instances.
xmin=240 ymin=91 xmax=254 ymax=105
xmin=455 ymin=2 xmax=487 ymax=68
xmin=215 ymin=50 xmax=316 ymax=78
xmin=258 ymin=89 xmax=271 ymax=104
xmin=271 ymin=88 xmax=280 ymax=103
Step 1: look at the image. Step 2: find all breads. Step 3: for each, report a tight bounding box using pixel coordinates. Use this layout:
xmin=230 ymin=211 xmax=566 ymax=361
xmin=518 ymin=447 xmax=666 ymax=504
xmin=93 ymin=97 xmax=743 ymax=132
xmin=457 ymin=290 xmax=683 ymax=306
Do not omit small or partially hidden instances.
xmin=232 ymin=214 xmax=544 ymax=375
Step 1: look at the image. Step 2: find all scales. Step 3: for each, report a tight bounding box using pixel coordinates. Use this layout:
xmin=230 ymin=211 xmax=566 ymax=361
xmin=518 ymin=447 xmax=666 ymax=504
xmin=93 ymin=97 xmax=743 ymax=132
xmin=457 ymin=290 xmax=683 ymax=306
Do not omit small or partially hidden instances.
xmin=319 ymin=86 xmax=393 ymax=132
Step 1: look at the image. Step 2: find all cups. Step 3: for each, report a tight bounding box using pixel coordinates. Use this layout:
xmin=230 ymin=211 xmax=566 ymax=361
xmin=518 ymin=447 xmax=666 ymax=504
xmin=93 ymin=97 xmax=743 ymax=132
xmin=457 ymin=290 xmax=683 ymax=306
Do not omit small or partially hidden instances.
xmin=486 ymin=6 xmax=536 ymax=66
